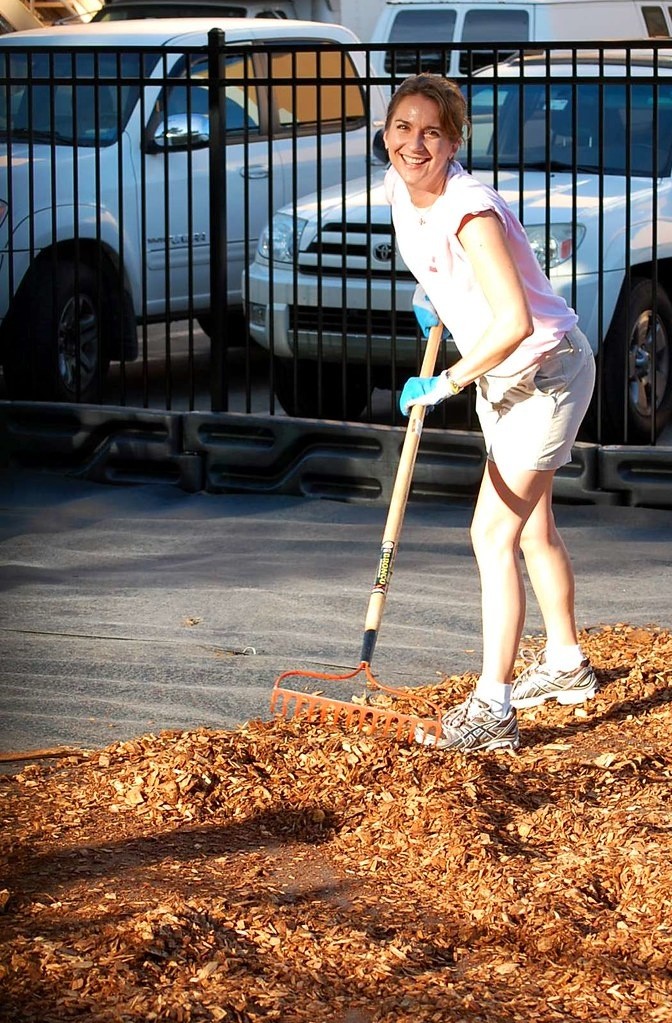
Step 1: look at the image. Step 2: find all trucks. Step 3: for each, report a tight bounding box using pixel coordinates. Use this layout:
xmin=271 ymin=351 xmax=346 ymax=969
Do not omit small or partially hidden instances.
xmin=88 ymin=0 xmax=388 ymax=56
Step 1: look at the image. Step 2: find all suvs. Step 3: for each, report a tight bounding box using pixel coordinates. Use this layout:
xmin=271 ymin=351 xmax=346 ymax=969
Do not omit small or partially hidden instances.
xmin=0 ymin=11 xmax=394 ymax=407
xmin=243 ymin=48 xmax=672 ymax=445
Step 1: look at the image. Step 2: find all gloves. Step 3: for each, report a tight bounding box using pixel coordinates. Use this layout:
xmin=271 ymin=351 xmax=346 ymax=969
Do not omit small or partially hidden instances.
xmin=399 ymin=369 xmax=464 ymax=417
xmin=412 ymin=280 xmax=452 ymax=341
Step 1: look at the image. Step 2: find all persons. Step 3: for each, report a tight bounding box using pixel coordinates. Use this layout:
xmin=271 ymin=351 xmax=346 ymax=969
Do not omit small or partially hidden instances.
xmin=383 ymin=72 xmax=600 ymax=752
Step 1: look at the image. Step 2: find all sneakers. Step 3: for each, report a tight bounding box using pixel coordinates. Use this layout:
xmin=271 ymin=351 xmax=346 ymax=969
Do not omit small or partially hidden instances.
xmin=414 ymin=692 xmax=519 ymax=751
xmin=509 ymin=648 xmax=600 ymax=710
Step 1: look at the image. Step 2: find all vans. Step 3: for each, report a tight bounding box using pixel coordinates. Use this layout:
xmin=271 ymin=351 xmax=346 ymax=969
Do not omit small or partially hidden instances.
xmin=363 ymin=0 xmax=672 ymax=110
